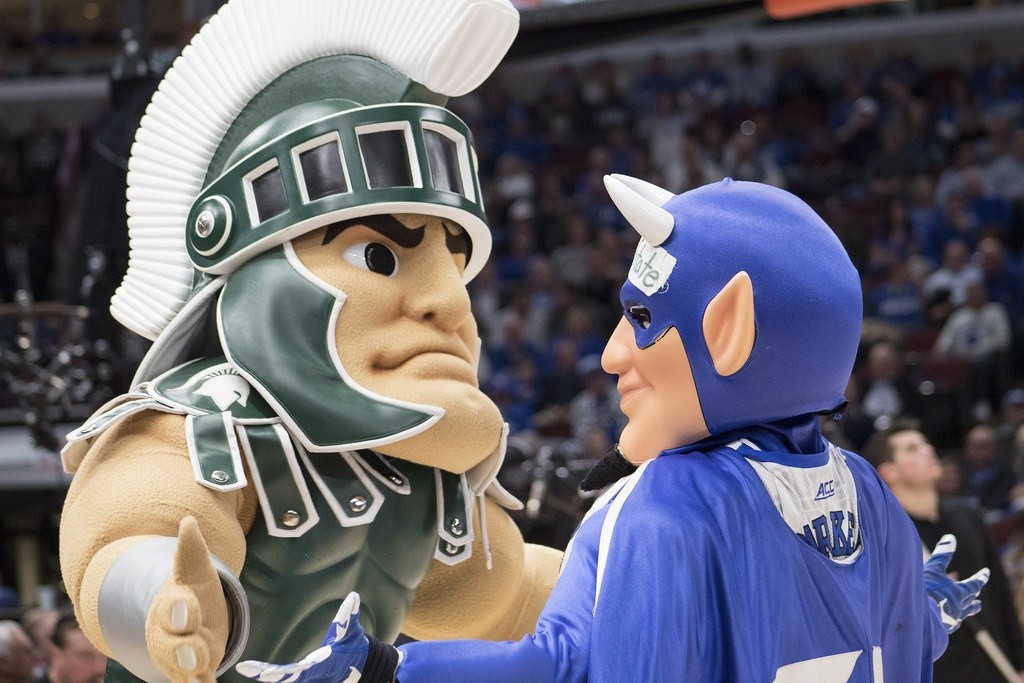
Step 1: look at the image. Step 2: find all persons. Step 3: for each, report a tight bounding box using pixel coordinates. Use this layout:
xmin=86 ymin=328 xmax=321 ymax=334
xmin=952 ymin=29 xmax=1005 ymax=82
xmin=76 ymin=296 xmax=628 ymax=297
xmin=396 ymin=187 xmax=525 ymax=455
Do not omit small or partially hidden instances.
xmin=0 ymin=1 xmax=1024 ymax=683
xmin=58 ymin=0 xmax=566 ymax=683
xmin=234 ymin=172 xmax=989 ymax=683
